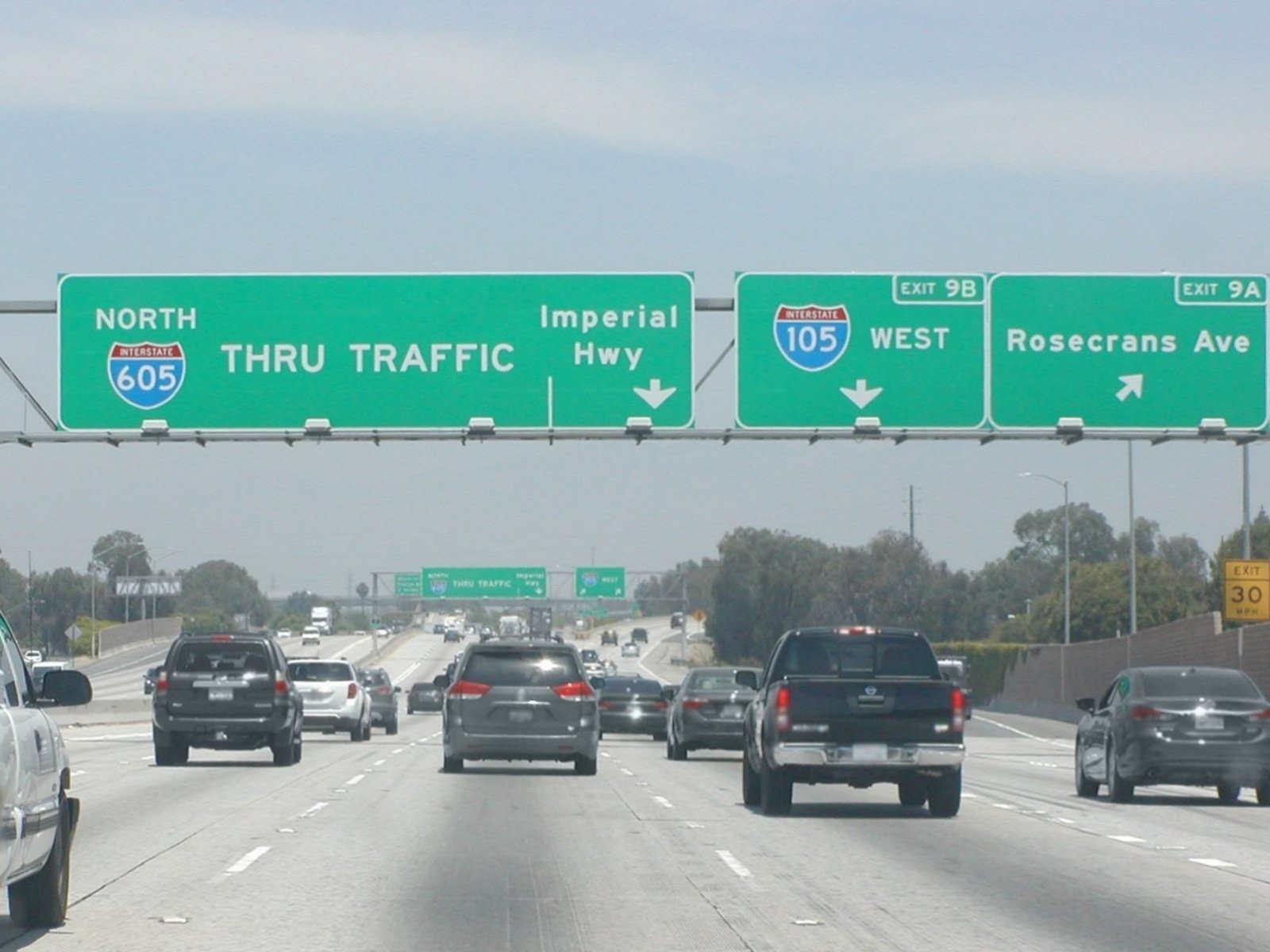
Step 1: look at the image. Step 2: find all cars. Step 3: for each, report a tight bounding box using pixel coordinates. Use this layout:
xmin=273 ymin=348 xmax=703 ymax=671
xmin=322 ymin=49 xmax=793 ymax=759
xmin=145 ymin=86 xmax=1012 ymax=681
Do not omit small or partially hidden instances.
xmin=405 ymin=619 xmax=618 ymax=715
xmin=0 ymin=607 xmax=92 ymax=931
xmin=624 ymin=629 xmax=647 ymax=658
xmin=1074 ymin=666 xmax=1270 ymax=806
xmin=598 ymin=677 xmax=676 ymax=743
xmin=661 ymin=665 xmax=763 ymax=762
xmin=279 ymin=629 xmax=292 ymax=638
xmin=300 ymin=604 xmax=332 ymax=644
xmin=349 ymin=618 xmax=407 ymax=635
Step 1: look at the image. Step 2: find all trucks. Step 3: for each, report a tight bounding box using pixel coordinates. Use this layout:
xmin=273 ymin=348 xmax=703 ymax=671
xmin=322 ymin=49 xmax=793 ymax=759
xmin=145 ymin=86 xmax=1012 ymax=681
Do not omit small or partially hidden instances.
xmin=936 ymin=655 xmax=977 ymax=719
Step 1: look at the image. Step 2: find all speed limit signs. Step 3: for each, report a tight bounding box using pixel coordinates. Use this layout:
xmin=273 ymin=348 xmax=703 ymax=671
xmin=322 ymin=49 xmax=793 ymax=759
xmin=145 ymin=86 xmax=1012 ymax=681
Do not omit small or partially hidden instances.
xmin=1225 ymin=558 xmax=1270 ymax=621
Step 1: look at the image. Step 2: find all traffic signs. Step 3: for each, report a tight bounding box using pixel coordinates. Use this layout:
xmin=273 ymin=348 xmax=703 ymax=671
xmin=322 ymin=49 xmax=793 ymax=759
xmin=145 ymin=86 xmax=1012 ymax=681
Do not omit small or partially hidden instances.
xmin=573 ymin=567 xmax=624 ymax=601
xmin=53 ymin=275 xmax=693 ymax=434
xmin=986 ymin=272 xmax=1268 ymax=432
xmin=731 ymin=270 xmax=987 ymax=432
xmin=394 ymin=574 xmax=421 ymax=597
xmin=420 ymin=568 xmax=548 ymax=601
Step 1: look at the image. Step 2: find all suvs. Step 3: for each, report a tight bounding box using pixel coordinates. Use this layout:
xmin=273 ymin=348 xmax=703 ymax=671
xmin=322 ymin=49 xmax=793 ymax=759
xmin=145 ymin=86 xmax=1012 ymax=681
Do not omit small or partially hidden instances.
xmin=671 ymin=611 xmax=683 ymax=630
xmin=144 ymin=632 xmax=402 ymax=768
xmin=432 ymin=633 xmax=605 ymax=778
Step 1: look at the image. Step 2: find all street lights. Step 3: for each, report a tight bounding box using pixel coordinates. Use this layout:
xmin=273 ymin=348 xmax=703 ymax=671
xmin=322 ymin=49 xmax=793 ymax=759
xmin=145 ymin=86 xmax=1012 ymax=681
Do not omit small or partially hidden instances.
xmin=1018 ymin=470 xmax=1071 ymax=640
xmin=89 ymin=536 xmax=183 ymax=659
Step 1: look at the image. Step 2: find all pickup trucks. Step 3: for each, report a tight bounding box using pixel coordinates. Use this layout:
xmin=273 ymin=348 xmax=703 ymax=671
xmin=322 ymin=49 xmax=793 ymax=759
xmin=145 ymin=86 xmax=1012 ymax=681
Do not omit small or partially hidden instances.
xmin=735 ymin=627 xmax=963 ymax=815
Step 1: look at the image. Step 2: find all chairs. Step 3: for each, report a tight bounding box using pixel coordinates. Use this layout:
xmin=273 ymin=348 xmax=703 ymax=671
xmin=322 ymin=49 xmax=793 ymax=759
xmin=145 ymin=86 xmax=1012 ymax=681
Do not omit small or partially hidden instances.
xmin=878 ymin=645 xmax=926 ymax=675
xmin=193 ymin=655 xmax=212 ymax=669
xmin=243 ymin=655 xmax=269 ymax=671
